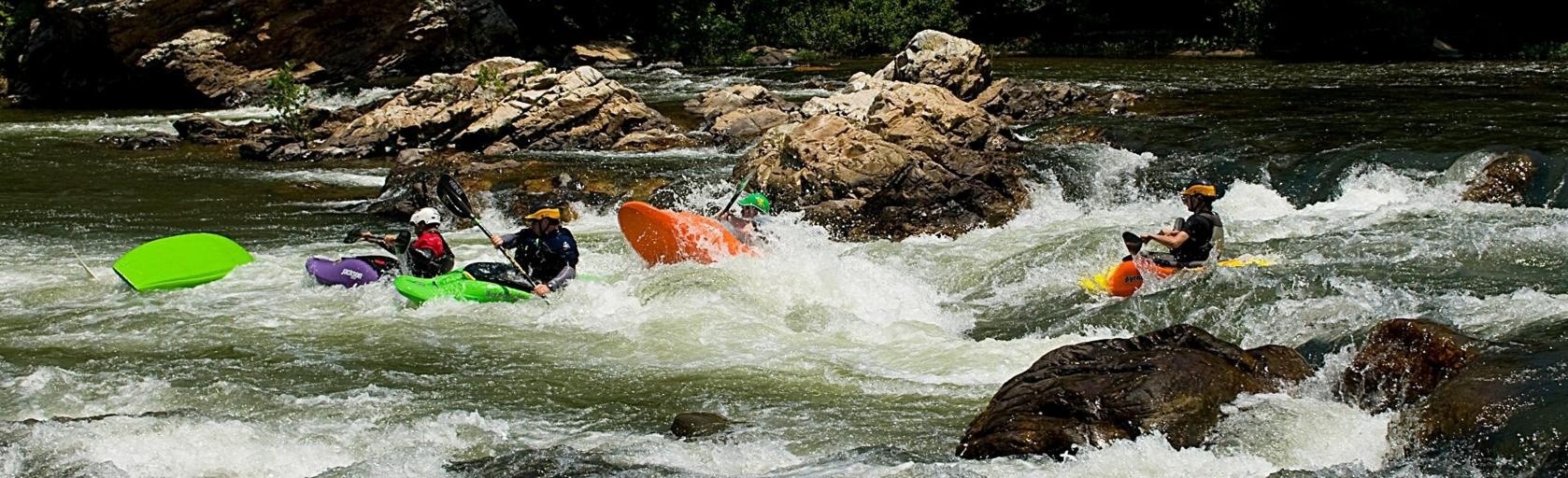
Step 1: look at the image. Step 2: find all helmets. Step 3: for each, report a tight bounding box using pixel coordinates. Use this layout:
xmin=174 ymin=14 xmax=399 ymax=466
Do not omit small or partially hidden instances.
xmin=736 ymin=191 xmax=771 ymax=214
xmin=523 ymin=201 xmax=561 ymax=221
xmin=1178 ymin=176 xmax=1218 ymax=198
xmin=409 ymin=206 xmax=442 ymax=227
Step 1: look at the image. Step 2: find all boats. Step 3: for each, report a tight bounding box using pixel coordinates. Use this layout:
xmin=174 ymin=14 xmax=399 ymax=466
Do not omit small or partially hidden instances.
xmin=110 ymin=233 xmax=252 ymax=291
xmin=617 ymin=201 xmax=759 ymax=264
xmin=306 ymin=204 xmax=575 ymax=300
xmin=1077 ymin=259 xmax=1275 ymax=297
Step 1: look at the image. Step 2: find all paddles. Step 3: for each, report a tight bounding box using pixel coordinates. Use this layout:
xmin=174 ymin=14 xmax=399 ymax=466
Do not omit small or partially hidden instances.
xmin=344 ymin=228 xmax=411 ymax=255
xmin=436 ymin=173 xmax=551 ymax=306
xmin=70 ymin=249 xmax=97 ymax=279
xmin=717 ymin=172 xmax=753 ymax=221
xmin=1123 ymin=217 xmax=1185 ymax=255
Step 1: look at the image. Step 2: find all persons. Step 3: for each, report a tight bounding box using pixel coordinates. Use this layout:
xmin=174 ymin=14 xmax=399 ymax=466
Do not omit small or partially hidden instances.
xmin=490 ymin=202 xmax=579 ymax=297
xmin=716 ymin=192 xmax=771 ymax=237
xmin=360 ymin=206 xmax=455 ymax=278
xmin=1140 ymin=178 xmax=1224 ymax=262
